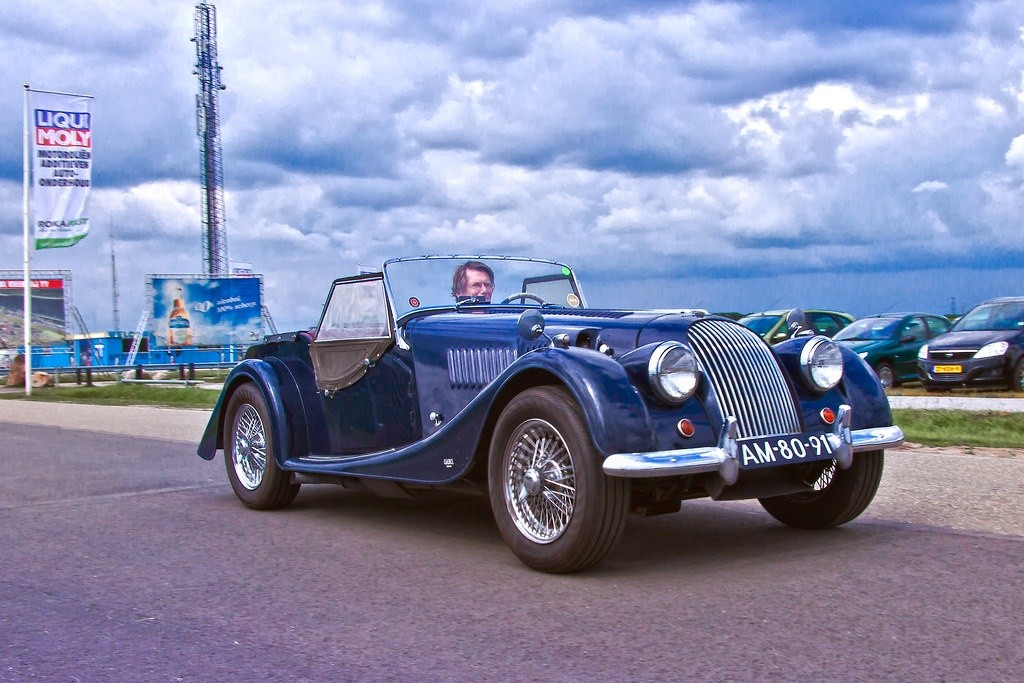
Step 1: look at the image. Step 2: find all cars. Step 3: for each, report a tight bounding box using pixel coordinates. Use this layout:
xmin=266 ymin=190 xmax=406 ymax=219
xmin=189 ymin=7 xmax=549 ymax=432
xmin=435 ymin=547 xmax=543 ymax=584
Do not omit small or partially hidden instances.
xmin=913 ymin=294 xmax=1024 ymax=392
xmin=831 ymin=312 xmax=951 ymax=388
xmin=194 ymin=254 xmax=904 ymax=574
xmin=941 ymin=312 xmax=997 ymax=332
xmin=736 ymin=309 xmax=856 ymax=346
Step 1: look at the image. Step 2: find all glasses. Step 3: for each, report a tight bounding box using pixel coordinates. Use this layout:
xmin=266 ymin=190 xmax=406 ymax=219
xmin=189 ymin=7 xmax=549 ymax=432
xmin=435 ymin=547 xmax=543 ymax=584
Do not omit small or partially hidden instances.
xmin=468 ymin=280 xmax=495 ymax=292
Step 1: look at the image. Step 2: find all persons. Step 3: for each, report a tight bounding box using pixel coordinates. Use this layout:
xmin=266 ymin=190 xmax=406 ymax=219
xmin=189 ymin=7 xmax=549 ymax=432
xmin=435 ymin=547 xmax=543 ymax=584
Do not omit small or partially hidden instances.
xmin=450 ymin=262 xmax=495 ymax=303
